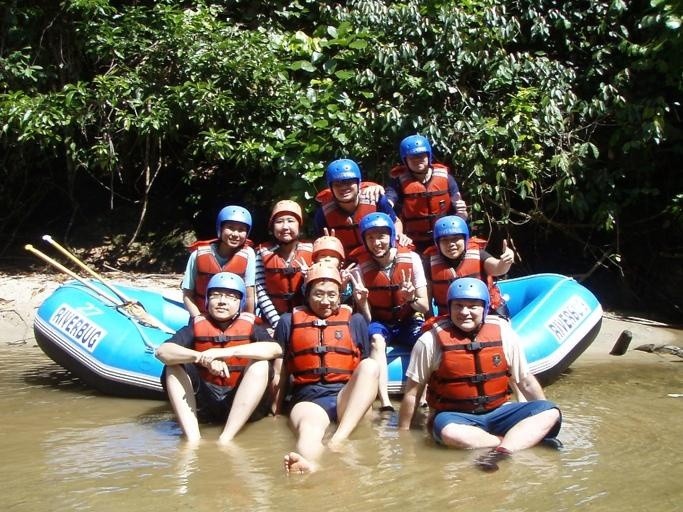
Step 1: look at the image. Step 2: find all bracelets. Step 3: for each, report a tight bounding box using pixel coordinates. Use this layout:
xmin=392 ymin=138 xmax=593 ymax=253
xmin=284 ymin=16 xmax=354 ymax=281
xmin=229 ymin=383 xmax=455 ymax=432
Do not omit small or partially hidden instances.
xmin=408 ymin=297 xmax=419 ymax=304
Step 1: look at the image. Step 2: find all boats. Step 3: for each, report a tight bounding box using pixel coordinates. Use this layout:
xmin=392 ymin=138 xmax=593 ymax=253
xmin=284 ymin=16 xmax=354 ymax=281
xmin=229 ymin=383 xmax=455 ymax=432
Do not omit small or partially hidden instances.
xmin=32 ymin=273 xmax=604 ymax=399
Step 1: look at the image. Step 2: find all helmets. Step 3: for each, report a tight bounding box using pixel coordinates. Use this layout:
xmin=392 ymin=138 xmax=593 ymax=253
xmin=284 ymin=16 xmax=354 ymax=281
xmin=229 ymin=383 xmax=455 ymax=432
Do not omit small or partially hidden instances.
xmin=327 ymin=159 xmax=361 ymax=194
xmin=216 ymin=205 xmax=252 ymax=239
xmin=400 ymin=134 xmax=432 ymax=169
xmin=434 ymin=215 xmax=469 ymax=254
xmin=447 ymin=277 xmax=490 ymax=324
xmin=359 ymin=212 xmax=396 ymax=251
xmin=269 ymin=199 xmax=303 ymax=229
xmin=304 ymin=262 xmax=342 ymax=297
xmin=204 ymin=272 xmax=246 ymax=317
xmin=310 ymin=236 xmax=345 ymax=262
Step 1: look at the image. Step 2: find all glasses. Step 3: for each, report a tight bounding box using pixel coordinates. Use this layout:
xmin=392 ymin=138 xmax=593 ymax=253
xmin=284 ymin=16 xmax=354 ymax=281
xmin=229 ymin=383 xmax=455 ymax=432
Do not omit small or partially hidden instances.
xmin=207 ymin=291 xmax=242 ymax=301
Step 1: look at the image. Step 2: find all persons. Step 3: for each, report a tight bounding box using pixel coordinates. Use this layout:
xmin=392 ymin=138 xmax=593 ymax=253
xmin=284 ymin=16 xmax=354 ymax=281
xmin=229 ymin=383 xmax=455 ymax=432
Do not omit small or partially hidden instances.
xmin=255 ymin=199 xmax=339 ymax=322
xmin=420 ymin=216 xmax=515 ymax=325
xmin=153 ymin=270 xmax=284 ymax=473
xmin=308 ymin=158 xmax=414 ymax=255
xmin=398 ymin=278 xmax=564 ymax=474
xmin=269 ymin=263 xmax=380 ymax=478
xmin=295 ymin=236 xmax=360 ymax=308
xmin=381 ymin=136 xmax=470 ymax=252
xmin=180 ymin=206 xmax=257 ymax=325
xmin=352 ymin=212 xmax=430 ymax=431
